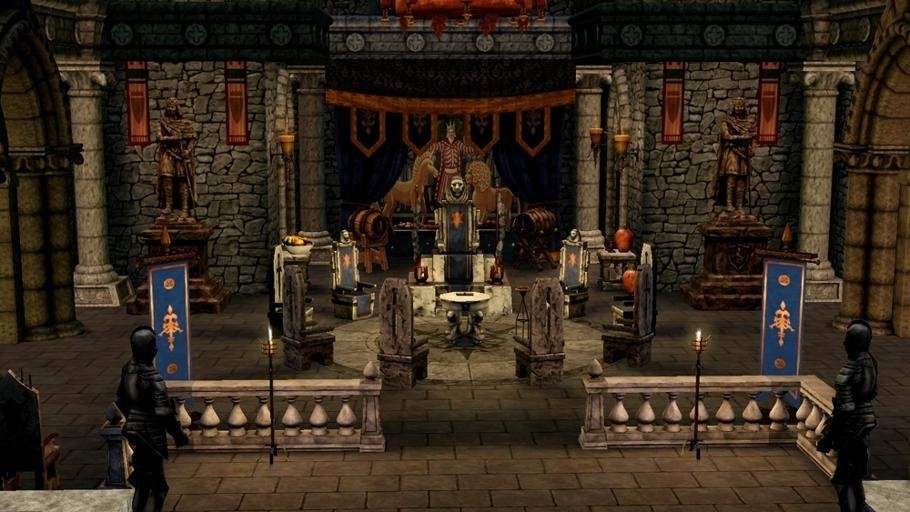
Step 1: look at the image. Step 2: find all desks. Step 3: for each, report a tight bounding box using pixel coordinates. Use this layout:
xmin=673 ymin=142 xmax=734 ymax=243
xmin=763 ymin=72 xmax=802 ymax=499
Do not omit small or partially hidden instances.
xmin=440 ymin=291 xmax=491 ymax=345
xmin=597 ymin=248 xmax=634 ymax=292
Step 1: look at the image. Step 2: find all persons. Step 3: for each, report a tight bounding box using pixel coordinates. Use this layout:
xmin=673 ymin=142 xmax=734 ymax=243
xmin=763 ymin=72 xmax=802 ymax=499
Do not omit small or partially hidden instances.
xmin=719 ymin=95 xmax=756 ymax=222
xmin=431 ymin=120 xmax=474 ymax=204
xmin=821 ymin=319 xmax=879 ymax=510
xmin=157 ymin=94 xmax=197 ymax=220
xmin=115 ymin=326 xmax=188 ymax=510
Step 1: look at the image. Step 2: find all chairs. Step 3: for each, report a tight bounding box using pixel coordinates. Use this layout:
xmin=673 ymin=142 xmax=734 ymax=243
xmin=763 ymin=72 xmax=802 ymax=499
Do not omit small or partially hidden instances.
xmin=330 ymin=230 xmax=377 ymax=321
xmin=280 ymin=266 xmax=336 ymax=372
xmin=602 ymin=264 xmax=654 ymax=368
xmin=559 ymin=229 xmax=591 ymax=318
xmin=512 ymin=277 xmax=564 ymax=386
xmin=376 ymin=278 xmax=431 ymax=389
xmin=270 ymin=244 xmax=314 ymax=336
xmin=611 ymin=242 xmax=658 ymax=330
xmin=433 ymin=202 xmax=486 ymax=284
xmin=0 ymin=366 xmax=63 ymax=490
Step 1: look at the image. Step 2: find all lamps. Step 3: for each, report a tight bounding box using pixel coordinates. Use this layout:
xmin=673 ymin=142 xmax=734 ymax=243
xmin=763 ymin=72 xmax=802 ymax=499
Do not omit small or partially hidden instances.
xmin=270 ymin=134 xmax=296 ymax=167
xmin=587 ymin=125 xmax=639 ymax=173
